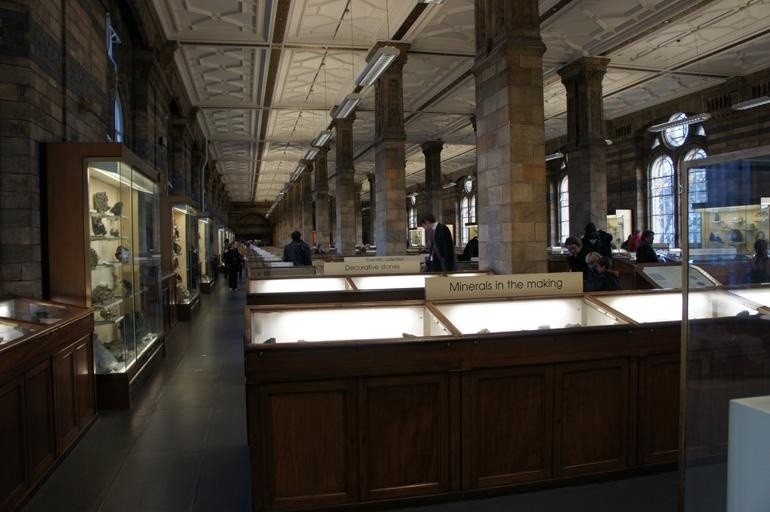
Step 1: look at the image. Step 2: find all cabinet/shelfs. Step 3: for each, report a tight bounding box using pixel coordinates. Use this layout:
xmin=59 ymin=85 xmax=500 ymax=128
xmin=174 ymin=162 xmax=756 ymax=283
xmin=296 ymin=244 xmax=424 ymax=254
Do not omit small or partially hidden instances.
xmin=191 ymin=212 xmax=218 ymax=296
xmin=47 ymin=143 xmax=169 ymax=405
xmin=692 ymin=201 xmax=769 ymax=258
xmin=158 ymin=192 xmax=201 ymax=323
xmin=213 ymin=223 xmax=236 ymax=271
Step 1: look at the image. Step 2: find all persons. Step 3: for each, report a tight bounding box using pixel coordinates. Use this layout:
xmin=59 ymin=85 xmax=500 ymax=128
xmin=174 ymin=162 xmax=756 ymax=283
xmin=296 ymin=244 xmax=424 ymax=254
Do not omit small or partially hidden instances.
xmin=564 ymin=221 xmax=622 ymax=291
xmin=314 ymin=238 xmax=335 ymax=254
xmin=282 ymin=230 xmax=312 ymax=265
xmin=732 ymin=243 xmax=752 ymax=285
xmin=636 ymin=230 xmax=658 ymax=263
xmin=423 ymin=214 xmax=456 ymax=272
xmin=628 ymin=229 xmax=640 ymax=251
xmin=420 ymin=219 xmax=439 ymax=272
xmin=621 ymin=235 xmax=631 ymax=250
xmin=462 ymin=236 xmax=478 ymax=261
xmin=211 ymin=236 xmax=257 ymax=293
xmin=745 ymin=239 xmax=770 ymax=284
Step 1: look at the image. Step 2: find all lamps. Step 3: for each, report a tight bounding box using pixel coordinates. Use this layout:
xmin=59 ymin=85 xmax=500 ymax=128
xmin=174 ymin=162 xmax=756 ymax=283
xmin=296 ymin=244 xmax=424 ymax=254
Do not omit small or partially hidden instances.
xmin=262 ymin=0 xmax=401 ymax=223
xmin=645 ymin=12 xmax=769 ymax=133
